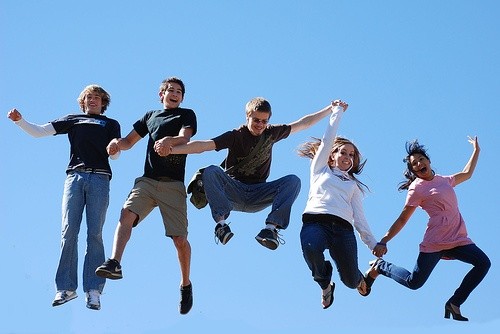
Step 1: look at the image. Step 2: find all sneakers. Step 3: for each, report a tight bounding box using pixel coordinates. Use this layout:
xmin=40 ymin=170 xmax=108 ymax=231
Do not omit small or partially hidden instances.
xmin=51 ymin=289 xmax=78 ymax=308
xmin=95 ymin=257 xmax=123 ymax=280
xmin=255 ymin=228 xmax=286 ymax=251
xmin=179 ymin=279 xmax=194 ymax=315
xmin=84 ymin=289 xmax=102 ymax=310
xmin=213 ymin=222 xmax=234 ymax=246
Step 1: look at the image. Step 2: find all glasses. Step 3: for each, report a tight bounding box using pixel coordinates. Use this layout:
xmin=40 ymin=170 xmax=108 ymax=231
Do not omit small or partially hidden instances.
xmin=250 ymin=115 xmax=270 ymax=125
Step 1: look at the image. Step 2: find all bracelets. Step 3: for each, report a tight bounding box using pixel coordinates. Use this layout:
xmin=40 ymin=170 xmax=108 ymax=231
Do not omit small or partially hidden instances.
xmin=376 ymin=242 xmax=386 ymax=246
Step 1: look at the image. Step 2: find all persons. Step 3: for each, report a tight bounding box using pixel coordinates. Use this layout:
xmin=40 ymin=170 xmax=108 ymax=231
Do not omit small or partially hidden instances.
xmin=95 ymin=77 xmax=198 ymax=315
xmin=296 ymin=100 xmax=388 ymax=308
xmin=155 ymin=96 xmax=343 ymax=251
xmin=6 ymin=84 xmax=121 ymax=311
xmin=364 ymin=136 xmax=491 ymax=321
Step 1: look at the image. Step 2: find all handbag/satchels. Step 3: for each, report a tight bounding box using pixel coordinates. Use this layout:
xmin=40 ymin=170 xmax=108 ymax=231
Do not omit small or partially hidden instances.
xmin=186 ymin=166 xmax=208 ymax=209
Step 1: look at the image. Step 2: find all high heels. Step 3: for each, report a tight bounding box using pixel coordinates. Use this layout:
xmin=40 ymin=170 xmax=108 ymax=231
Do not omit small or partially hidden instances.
xmin=364 ymin=258 xmax=383 ymax=288
xmin=444 ymin=300 xmax=469 ymax=321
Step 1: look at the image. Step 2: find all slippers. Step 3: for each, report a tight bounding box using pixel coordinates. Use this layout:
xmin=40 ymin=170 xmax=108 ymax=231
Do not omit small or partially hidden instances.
xmin=321 ymin=281 xmax=335 ymax=309
xmin=356 ymin=270 xmax=371 ymax=296
